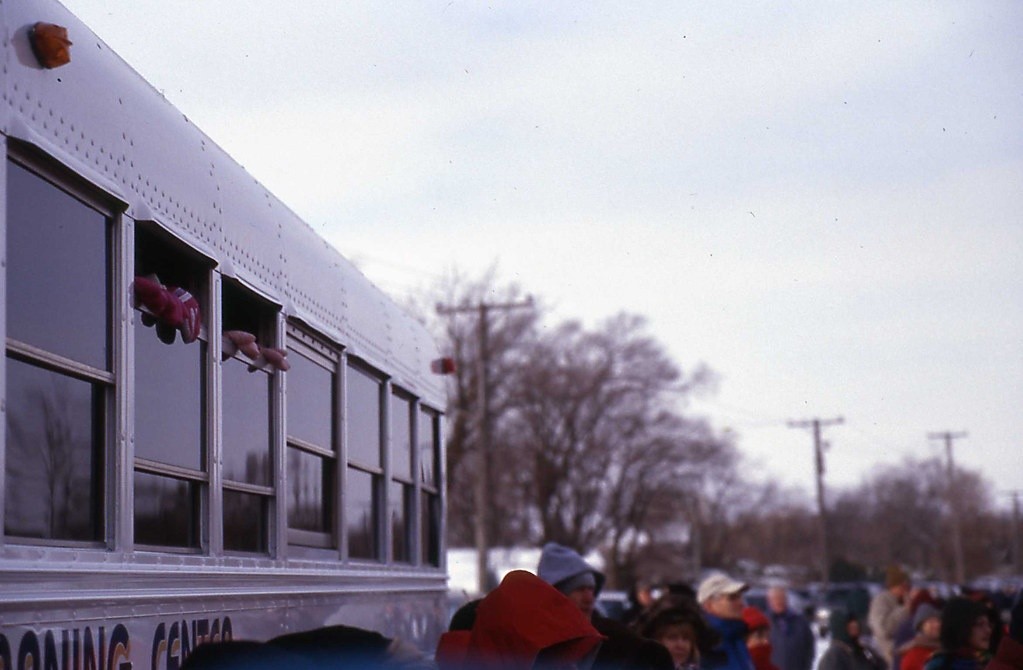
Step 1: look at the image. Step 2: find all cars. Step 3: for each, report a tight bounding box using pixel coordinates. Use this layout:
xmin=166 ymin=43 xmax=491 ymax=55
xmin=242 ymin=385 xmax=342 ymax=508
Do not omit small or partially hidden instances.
xmin=743 ymin=580 xmax=1020 ymax=645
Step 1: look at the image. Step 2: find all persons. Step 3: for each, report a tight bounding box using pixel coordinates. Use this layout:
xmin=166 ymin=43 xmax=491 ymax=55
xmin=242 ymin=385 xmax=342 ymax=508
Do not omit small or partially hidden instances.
xmin=179 ymin=543 xmax=1023 ymax=670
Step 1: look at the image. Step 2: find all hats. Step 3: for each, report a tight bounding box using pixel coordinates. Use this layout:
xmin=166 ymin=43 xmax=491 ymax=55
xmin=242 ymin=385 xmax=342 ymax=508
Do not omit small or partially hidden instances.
xmin=696 ymin=570 xmax=746 ymax=605
xmin=881 ymin=561 xmax=913 ymax=590
xmin=913 ymin=601 xmax=943 ymax=631
xmin=740 ymin=606 xmax=770 ymax=633
xmin=554 ymin=571 xmax=597 ymax=594
